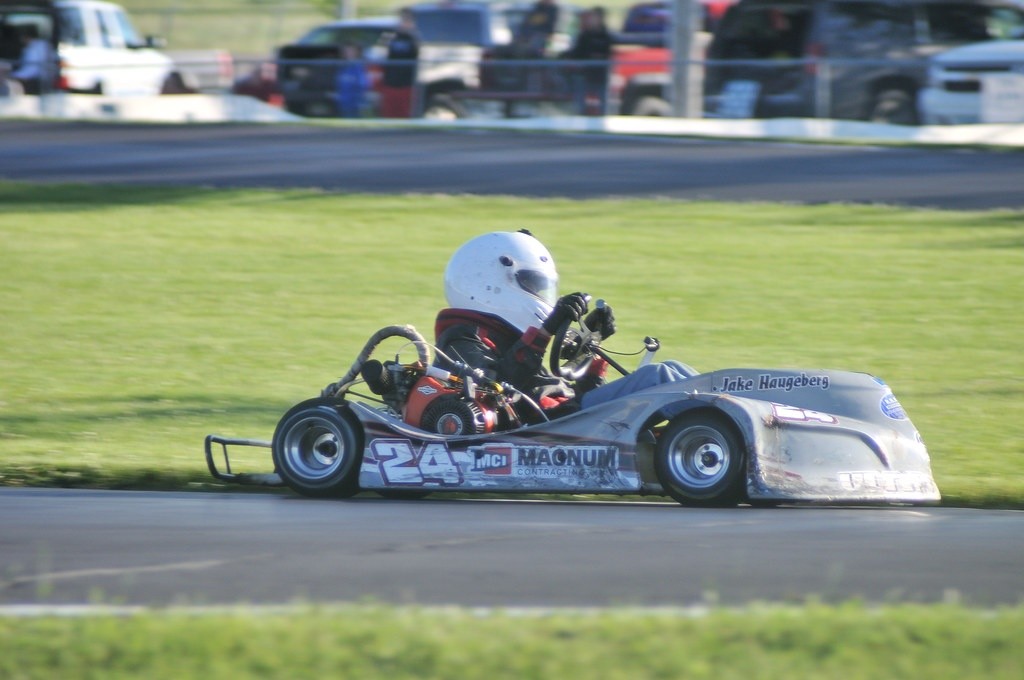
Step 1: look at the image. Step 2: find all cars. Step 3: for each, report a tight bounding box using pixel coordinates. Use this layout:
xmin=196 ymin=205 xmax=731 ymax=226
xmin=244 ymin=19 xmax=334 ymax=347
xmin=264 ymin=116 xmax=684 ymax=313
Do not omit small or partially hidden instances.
xmin=274 ymin=0 xmax=1024 ymax=135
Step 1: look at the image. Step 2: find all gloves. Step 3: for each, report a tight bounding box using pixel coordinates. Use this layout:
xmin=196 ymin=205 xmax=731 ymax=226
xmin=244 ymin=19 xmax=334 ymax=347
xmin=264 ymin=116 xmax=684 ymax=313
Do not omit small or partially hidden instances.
xmin=542 ymin=292 xmax=591 ymax=337
xmin=560 ymin=305 xmax=616 ymax=360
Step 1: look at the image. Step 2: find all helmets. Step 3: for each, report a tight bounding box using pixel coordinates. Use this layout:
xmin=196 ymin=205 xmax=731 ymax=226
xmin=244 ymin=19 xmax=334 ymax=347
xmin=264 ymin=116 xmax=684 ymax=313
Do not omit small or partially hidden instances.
xmin=443 ymin=232 xmax=558 ymax=334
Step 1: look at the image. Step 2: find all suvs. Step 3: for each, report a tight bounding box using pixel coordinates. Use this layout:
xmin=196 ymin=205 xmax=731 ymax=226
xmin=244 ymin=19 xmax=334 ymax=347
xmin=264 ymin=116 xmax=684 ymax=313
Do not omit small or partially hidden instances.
xmin=1 ymin=0 xmax=192 ymax=100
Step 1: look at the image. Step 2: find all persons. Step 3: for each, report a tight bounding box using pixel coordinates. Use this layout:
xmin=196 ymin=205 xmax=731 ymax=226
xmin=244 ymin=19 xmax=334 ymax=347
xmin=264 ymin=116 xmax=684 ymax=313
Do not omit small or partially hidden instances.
xmin=381 ymin=8 xmax=419 ymax=119
xmin=558 ymin=6 xmax=611 ymax=116
xmin=160 ymin=64 xmax=280 ymax=103
xmin=335 ymin=45 xmax=369 ymax=119
xmin=484 ymin=0 xmax=558 ymax=118
xmin=402 ymin=229 xmax=699 ymax=434
xmin=701 ymin=1 xmax=811 ymax=117
xmin=9 ymin=23 xmax=64 ymax=94
